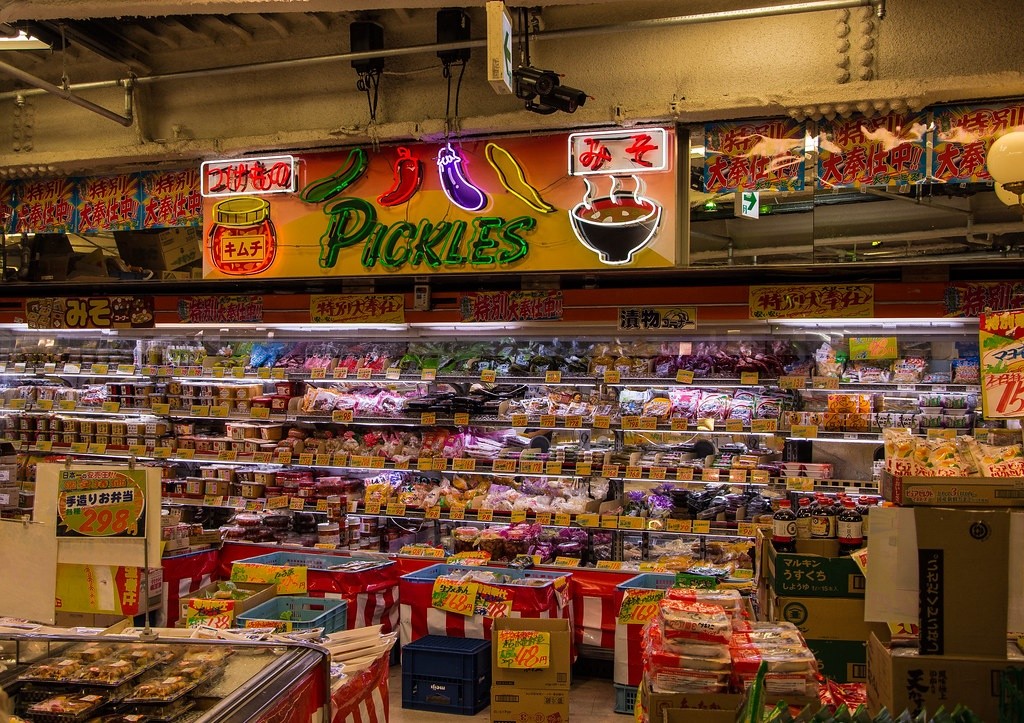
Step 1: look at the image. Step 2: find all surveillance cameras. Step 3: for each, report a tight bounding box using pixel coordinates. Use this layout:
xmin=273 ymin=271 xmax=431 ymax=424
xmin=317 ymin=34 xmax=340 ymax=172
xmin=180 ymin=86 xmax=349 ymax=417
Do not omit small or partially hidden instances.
xmin=540 ymin=84 xmax=588 ymax=113
xmin=515 ymin=67 xmax=554 ymax=96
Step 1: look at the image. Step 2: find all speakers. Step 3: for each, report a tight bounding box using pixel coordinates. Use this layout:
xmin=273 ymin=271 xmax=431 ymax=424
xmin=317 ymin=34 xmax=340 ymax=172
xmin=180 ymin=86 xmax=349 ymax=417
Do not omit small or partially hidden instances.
xmin=438 ymin=8 xmax=471 ymax=61
xmin=349 ymin=21 xmax=384 ymax=69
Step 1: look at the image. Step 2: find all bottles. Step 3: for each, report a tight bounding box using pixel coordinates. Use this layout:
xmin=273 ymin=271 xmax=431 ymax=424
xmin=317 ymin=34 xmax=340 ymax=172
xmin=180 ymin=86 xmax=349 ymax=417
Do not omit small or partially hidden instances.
xmin=837 ymin=492 xmax=861 ymax=551
xmin=812 ymin=491 xmax=836 ymax=539
xmin=773 ymin=500 xmax=797 ymax=554
xmin=796 ymin=497 xmax=812 ymax=538
xmin=858 ymin=496 xmax=879 ymax=540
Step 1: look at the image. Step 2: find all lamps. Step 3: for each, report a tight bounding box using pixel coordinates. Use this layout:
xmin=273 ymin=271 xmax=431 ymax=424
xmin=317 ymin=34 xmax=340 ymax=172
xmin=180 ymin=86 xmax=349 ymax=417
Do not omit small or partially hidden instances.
xmin=993 ymin=181 xmax=1024 ymax=208
xmin=987 ymin=132 xmax=1024 ymax=210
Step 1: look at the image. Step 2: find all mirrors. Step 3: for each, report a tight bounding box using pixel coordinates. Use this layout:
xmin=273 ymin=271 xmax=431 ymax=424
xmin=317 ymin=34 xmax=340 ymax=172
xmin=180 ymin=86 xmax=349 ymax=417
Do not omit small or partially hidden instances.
xmin=687 ymin=126 xmax=1024 ymax=266
xmin=0 ymin=226 xmax=204 ymax=284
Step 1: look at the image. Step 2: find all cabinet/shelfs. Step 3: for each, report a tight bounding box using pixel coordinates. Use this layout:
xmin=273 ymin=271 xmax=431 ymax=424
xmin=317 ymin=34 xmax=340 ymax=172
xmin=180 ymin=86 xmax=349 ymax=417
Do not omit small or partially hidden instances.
xmin=0 ymin=317 xmax=1024 ymax=574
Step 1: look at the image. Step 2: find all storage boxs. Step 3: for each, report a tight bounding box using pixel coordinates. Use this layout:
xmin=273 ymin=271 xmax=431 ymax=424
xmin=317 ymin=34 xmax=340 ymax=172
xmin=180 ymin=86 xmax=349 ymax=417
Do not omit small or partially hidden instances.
xmin=662 ymin=707 xmax=736 ymax=723
xmin=399 ymin=563 xmax=574 ymax=589
xmin=178 ymin=580 xmax=278 ymax=624
xmin=746 ymin=686 xmax=819 ymax=717
xmin=849 ymin=336 xmax=897 ymax=361
xmin=613 ymin=683 xmax=638 ymax=715
xmin=866 ymin=628 xmax=1024 ymax=723
xmin=0 ymin=464 xmax=17 ymax=487
xmin=230 ymin=550 xmax=397 ymax=573
xmin=0 ymin=487 xmax=19 ymax=511
xmin=0 ymin=442 xmax=17 ymax=464
xmin=490 ymin=617 xmax=571 ymax=690
xmin=753 ymin=523 xmax=893 ymax=686
xmin=863 ymin=505 xmax=1012 ymax=659
xmin=28 ymin=226 xmax=203 ymax=281
xmin=641 ymin=670 xmax=743 ymax=723
xmin=880 ymin=467 xmax=1024 ymax=507
xmin=490 ymin=686 xmax=570 ymax=723
xmin=402 ymin=634 xmax=491 ymax=716
xmin=616 ymin=572 xmax=752 ymax=594
xmin=235 ymin=595 xmax=348 ymax=634
xmin=159 ymin=515 xmax=220 ymax=557
